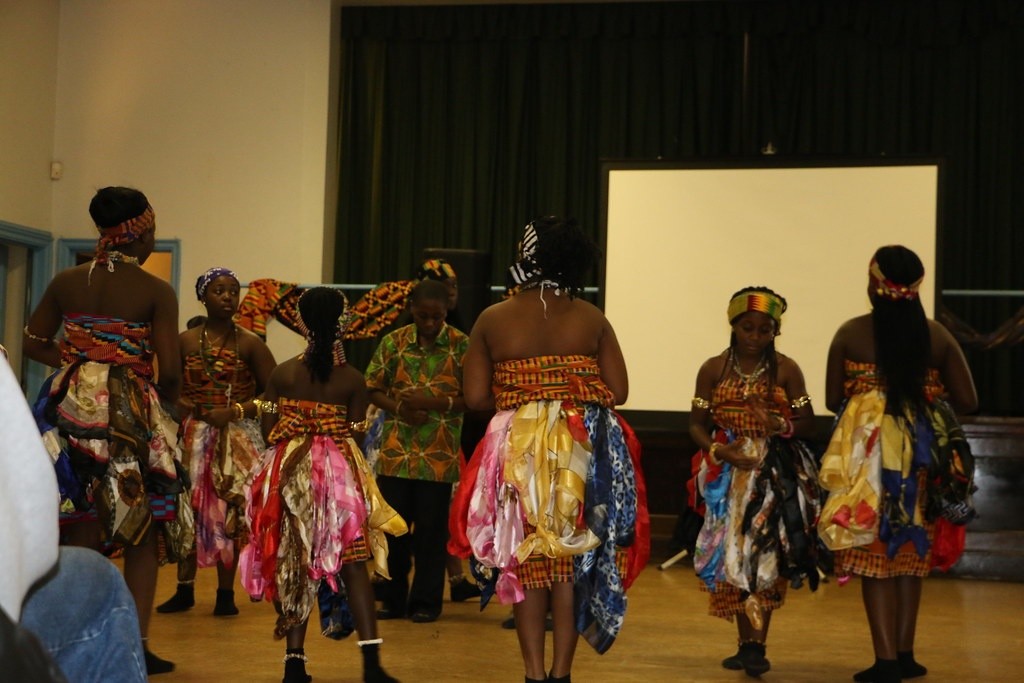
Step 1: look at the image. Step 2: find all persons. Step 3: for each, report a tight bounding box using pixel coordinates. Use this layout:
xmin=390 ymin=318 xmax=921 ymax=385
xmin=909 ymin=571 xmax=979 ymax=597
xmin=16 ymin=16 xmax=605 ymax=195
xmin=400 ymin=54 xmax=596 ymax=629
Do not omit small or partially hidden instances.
xmin=235 ymin=285 xmax=408 ymax=683
xmin=448 ymin=216 xmax=650 ymax=683
xmin=154 ymin=268 xmax=277 ymax=617
xmin=364 ymin=279 xmax=470 ymax=622
xmin=23 ymin=185 xmax=195 ymax=675
xmin=0 ymin=344 xmax=148 ymax=683
xmin=370 ymin=258 xmax=482 ymax=602
xmin=817 ymin=244 xmax=978 ymax=683
xmin=687 ymin=286 xmax=835 ymax=676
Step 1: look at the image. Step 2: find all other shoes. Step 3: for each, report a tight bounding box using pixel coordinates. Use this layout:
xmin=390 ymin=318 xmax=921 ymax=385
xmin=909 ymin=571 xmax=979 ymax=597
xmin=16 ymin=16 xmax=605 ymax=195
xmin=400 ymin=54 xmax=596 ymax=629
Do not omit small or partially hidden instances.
xmin=376 ymin=607 xmax=403 ymax=619
xmin=413 ymin=607 xmax=436 ymax=622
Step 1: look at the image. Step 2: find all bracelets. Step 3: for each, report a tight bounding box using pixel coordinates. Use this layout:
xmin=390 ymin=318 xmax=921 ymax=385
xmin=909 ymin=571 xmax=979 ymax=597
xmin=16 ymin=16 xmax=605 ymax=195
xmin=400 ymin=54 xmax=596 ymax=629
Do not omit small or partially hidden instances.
xmin=232 ymin=403 xmax=244 ymax=422
xmin=396 ymin=401 xmax=402 ymax=414
xmin=445 ymin=396 xmax=452 ymax=414
xmin=709 ymin=443 xmax=723 ymax=465
xmin=774 ymin=417 xmax=793 ymax=437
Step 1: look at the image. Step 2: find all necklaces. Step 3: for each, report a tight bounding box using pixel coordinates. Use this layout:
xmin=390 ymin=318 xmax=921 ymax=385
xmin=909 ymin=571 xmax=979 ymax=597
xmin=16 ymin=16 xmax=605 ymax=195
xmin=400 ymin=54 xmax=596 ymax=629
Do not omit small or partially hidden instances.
xmin=730 ymin=349 xmax=769 ymax=400
xmin=199 ymin=320 xmax=239 ymax=386
xmin=93 ymin=251 xmax=138 ymax=264
xmin=515 ymin=280 xmax=551 ymax=292
